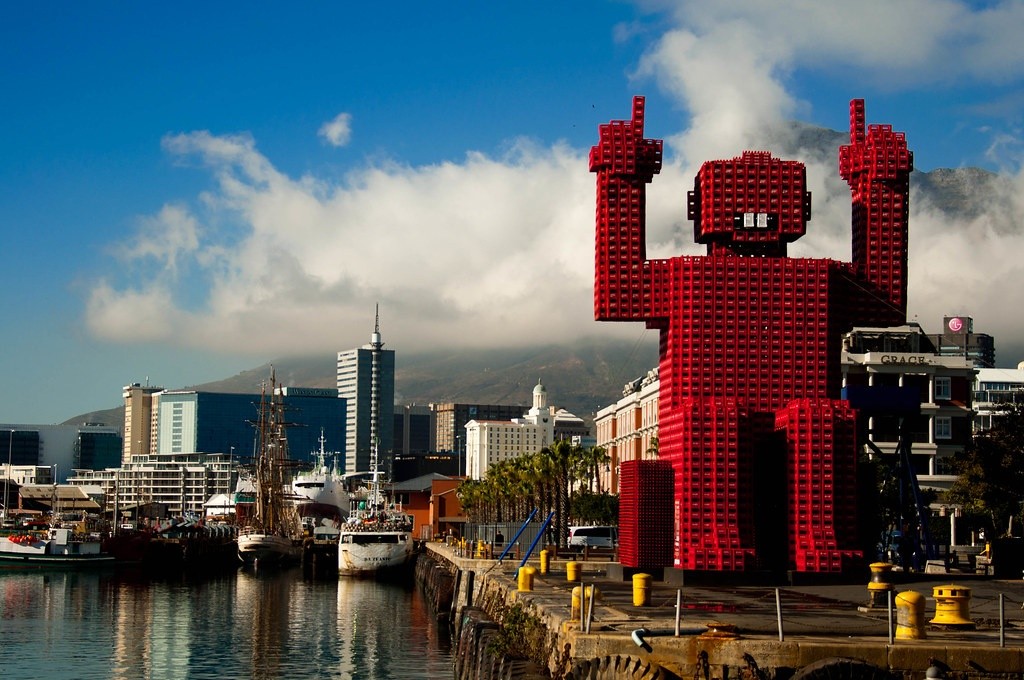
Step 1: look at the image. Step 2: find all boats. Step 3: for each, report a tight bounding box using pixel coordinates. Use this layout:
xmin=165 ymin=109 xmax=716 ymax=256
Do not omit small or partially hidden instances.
xmin=0 ymin=528 xmax=116 ymax=571
xmin=338 ymin=437 xmax=414 ymax=577
xmin=294 ymin=428 xmax=350 ymax=522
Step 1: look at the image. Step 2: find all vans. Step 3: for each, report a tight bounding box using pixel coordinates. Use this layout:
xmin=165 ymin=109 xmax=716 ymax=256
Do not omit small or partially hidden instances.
xmin=566 ymin=525 xmax=618 ymax=551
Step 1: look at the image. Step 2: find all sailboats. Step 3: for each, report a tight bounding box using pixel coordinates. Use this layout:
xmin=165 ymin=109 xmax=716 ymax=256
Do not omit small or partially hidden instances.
xmin=237 ymin=367 xmax=303 ymax=576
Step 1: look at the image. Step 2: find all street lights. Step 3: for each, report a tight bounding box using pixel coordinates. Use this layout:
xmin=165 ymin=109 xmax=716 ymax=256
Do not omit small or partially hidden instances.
xmin=7 ymin=429 xmax=15 ymax=518
xmin=228 ymin=446 xmax=236 ymax=519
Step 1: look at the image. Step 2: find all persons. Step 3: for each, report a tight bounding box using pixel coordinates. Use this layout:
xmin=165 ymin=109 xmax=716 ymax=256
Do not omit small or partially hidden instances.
xmin=240 ymin=525 xmax=282 ymax=536
xmin=10 ymin=528 xmax=29 ymax=537
xmin=590 ymin=96 xmax=914 ymax=574
xmin=347 ymin=521 xmax=411 ymax=532
xmin=137 ymin=516 xmax=160 ymax=534
xmin=495 ymin=531 xmax=504 ymax=547
xmin=73 ymin=534 xmax=91 ymax=540
